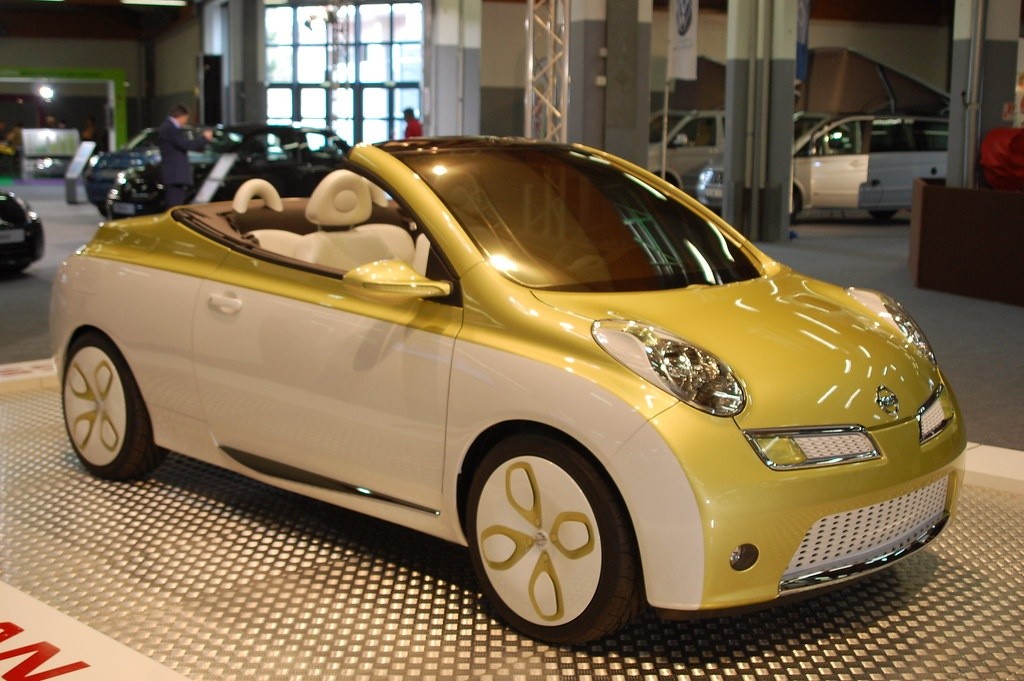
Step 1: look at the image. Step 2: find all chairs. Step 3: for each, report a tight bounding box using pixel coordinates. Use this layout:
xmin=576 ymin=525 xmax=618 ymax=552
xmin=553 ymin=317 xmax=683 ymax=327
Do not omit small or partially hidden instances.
xmin=233 ymin=168 xmax=431 ymax=279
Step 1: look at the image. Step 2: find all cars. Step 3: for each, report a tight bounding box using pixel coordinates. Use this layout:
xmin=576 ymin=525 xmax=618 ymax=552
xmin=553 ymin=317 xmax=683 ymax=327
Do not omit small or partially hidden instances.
xmin=649 ymin=108 xmax=726 ymax=196
xmin=84 ymin=126 xmax=207 ymax=219
xmin=108 ymin=127 xmax=353 ymax=224
xmin=0 ymin=189 xmax=45 ymax=278
xmin=45 ymin=136 xmax=964 ymax=646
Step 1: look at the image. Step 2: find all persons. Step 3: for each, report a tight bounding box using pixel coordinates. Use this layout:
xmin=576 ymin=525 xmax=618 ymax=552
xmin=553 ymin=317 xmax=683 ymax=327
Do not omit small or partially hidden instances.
xmin=0 ymin=116 xmax=99 ymax=178
xmin=404 ymin=109 xmax=422 ymax=138
xmin=160 ymin=105 xmax=212 ymax=214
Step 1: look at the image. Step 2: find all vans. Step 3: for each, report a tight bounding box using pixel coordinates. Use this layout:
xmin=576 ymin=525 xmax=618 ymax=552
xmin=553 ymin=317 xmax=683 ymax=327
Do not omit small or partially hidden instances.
xmin=696 ymin=110 xmax=948 ymax=221
